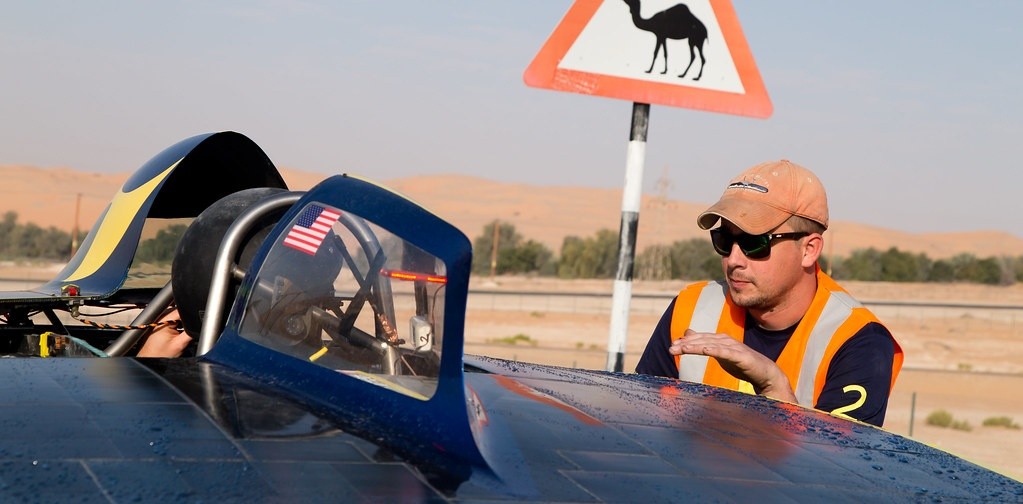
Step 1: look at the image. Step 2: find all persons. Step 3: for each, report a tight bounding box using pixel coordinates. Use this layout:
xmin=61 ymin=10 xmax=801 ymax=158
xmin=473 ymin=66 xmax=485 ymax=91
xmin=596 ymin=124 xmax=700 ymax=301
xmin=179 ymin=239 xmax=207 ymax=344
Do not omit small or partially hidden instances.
xmin=136 ymin=187 xmax=343 ymax=360
xmin=635 ymin=161 xmax=905 ymax=431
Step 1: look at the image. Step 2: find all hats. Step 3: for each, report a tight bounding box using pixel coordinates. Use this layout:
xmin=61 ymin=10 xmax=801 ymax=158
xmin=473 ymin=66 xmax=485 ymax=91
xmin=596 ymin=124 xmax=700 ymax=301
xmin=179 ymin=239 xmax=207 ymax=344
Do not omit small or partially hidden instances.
xmin=697 ymin=159 xmax=829 ymax=236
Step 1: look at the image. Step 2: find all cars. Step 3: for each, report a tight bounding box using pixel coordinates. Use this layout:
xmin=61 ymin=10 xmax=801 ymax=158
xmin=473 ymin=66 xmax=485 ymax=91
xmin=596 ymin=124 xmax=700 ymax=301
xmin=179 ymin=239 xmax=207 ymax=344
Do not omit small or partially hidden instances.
xmin=3 ymin=132 xmax=1023 ymax=503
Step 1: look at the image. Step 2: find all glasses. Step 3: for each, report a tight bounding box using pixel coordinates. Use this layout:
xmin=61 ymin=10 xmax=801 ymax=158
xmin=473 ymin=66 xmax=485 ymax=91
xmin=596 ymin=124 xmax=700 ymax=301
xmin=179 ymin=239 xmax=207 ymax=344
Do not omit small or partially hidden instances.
xmin=710 ymin=225 xmax=810 ymax=258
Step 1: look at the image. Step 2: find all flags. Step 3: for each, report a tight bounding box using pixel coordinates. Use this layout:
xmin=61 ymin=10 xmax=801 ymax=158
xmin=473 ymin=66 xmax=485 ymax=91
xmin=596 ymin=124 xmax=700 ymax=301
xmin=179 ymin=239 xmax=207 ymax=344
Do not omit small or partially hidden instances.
xmin=284 ymin=201 xmax=341 ymax=256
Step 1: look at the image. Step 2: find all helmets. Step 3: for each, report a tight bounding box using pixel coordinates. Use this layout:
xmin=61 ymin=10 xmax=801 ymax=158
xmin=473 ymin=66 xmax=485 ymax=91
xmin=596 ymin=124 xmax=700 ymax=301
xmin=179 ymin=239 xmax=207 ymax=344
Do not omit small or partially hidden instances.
xmin=171 ymin=187 xmax=345 ymax=339
